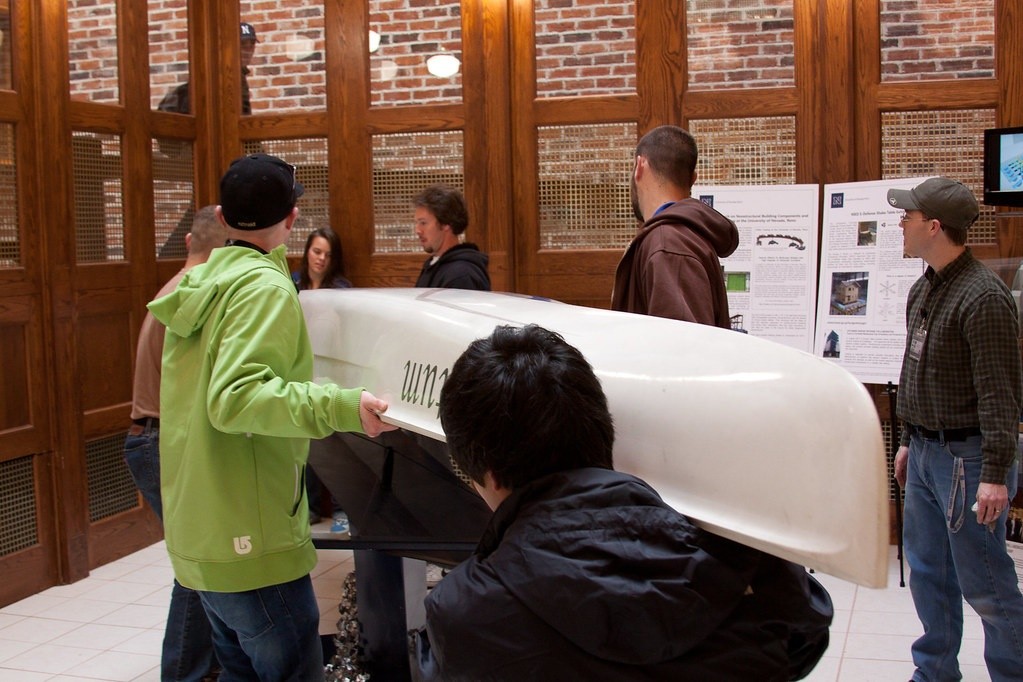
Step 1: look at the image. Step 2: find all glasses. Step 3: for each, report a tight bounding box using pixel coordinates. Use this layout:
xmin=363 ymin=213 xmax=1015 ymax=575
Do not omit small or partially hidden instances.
xmin=901 ymin=213 xmax=944 ymax=231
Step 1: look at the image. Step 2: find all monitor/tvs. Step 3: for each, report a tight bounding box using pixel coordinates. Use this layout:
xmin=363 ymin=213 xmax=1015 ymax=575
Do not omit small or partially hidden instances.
xmin=984 ymin=125 xmax=1023 ymax=208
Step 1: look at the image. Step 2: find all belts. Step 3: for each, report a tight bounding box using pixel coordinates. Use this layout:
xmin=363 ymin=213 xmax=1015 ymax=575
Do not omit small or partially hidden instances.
xmin=912 ymin=425 xmax=982 ymax=443
xmin=133 ymin=416 xmax=160 ymax=428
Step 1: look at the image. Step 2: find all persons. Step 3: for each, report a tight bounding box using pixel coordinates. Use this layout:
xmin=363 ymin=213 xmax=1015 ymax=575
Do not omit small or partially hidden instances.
xmin=424 ymin=322 xmax=834 ymax=681
xmin=146 ymin=153 xmax=399 ymax=681
xmin=157 ymin=22 xmax=261 ymax=259
xmin=611 ymin=125 xmax=740 ymax=330
xmin=412 ymin=186 xmax=492 ymax=290
xmin=886 ymin=176 xmax=1023 ymax=682
xmin=125 ymin=205 xmax=225 ymax=517
xmin=294 ymin=228 xmax=355 ymax=535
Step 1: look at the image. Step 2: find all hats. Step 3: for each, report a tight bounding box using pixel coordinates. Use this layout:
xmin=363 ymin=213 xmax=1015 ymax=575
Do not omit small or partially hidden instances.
xmin=218 ymin=153 xmax=304 ymax=231
xmin=887 ymin=177 xmax=979 ymax=230
xmin=240 ymin=22 xmax=260 ymax=43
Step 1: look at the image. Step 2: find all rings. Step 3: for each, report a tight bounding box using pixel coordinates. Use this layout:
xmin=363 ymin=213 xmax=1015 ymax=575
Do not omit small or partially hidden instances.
xmin=996 ymin=511 xmax=1002 ymax=513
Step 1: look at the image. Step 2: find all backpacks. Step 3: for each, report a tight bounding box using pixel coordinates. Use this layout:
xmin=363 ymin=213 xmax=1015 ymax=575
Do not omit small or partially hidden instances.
xmin=155 ymin=83 xmax=189 ymax=158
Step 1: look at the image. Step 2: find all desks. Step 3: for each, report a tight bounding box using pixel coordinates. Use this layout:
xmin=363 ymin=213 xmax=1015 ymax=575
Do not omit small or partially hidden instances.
xmin=309 ymin=504 xmax=480 ymax=682
